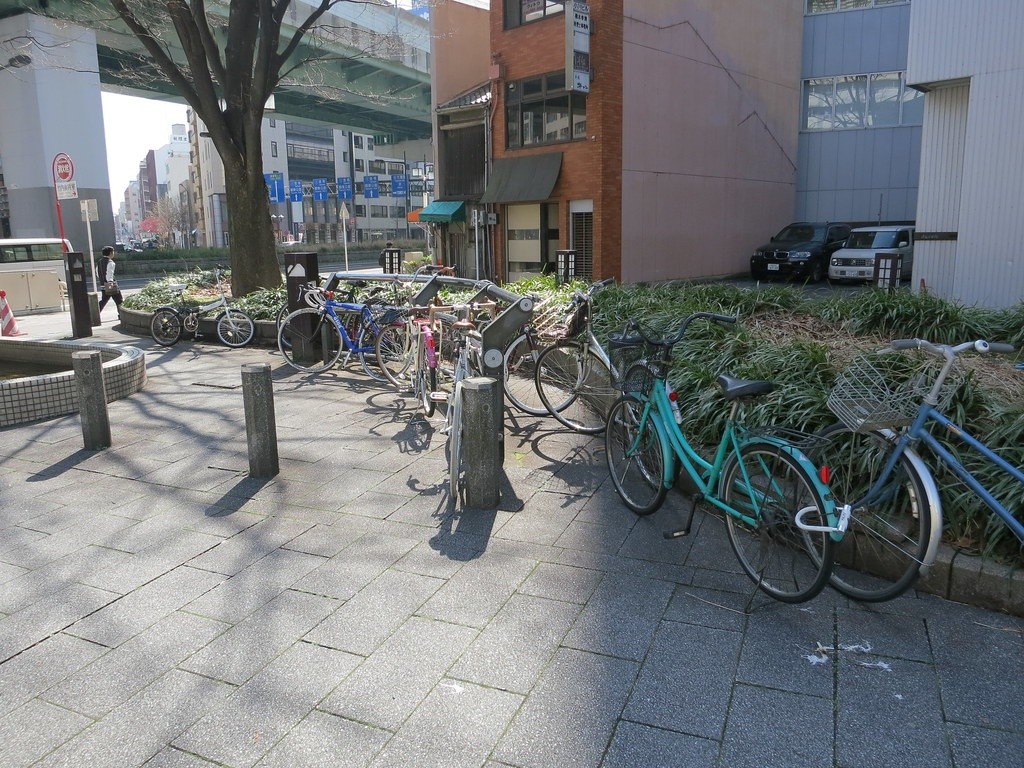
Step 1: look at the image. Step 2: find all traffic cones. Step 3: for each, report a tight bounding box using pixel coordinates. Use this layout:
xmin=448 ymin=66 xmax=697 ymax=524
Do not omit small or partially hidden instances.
xmin=0 ymin=290 xmax=27 ymax=336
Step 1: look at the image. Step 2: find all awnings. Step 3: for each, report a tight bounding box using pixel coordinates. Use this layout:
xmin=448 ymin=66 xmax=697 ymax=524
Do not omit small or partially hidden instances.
xmin=418 ymin=201 xmax=466 ymax=232
xmin=479 ymin=152 xmax=563 ymax=204
xmin=407 ymin=208 xmax=428 ymax=234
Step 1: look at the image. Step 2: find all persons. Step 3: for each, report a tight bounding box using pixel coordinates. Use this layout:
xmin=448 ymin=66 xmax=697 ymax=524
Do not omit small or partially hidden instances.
xmin=379 ymin=241 xmax=393 ymax=274
xmin=95 ymin=246 xmax=123 ymax=312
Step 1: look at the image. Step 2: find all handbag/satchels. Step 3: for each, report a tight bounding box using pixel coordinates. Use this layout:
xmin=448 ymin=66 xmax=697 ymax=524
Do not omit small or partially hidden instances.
xmin=103 ymin=280 xmax=119 ymax=295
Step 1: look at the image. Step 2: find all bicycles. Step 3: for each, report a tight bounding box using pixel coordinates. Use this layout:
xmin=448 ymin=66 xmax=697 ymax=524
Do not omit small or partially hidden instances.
xmin=478 ymin=283 xmax=586 ymax=416
xmin=534 ymin=278 xmax=683 ymax=490
xmin=277 ymin=286 xmax=414 ymax=382
xmin=149 ymin=262 xmax=256 ymax=348
xmin=425 ymin=297 xmax=495 ymax=498
xmin=605 ymin=311 xmax=852 ymax=613
xmin=275 ymin=274 xmax=400 ymax=366
xmin=382 ymin=264 xmax=443 ymax=419
xmin=793 ymin=338 xmax=1024 ymax=604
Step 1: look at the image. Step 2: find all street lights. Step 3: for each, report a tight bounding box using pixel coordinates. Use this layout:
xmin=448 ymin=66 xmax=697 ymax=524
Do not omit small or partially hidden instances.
xmin=375 ymin=151 xmax=409 ymax=239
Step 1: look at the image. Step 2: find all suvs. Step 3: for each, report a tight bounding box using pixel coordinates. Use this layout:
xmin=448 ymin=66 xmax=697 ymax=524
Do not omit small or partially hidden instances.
xmin=828 ymin=225 xmax=915 ymax=285
xmin=750 ymin=222 xmax=851 ymax=283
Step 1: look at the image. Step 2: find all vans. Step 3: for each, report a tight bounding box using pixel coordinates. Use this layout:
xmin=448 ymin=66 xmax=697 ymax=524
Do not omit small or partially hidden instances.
xmin=0 ymin=238 xmax=75 ymax=282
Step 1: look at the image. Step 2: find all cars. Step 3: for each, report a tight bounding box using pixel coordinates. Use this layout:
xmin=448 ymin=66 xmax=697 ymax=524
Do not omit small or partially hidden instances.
xmin=116 ymin=242 xmax=124 ymax=250
xmin=133 ymin=242 xmax=141 ymax=249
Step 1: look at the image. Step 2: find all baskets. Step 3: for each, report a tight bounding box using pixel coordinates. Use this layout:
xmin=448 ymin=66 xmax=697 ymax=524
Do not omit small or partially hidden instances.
xmin=826 ymin=354 xmax=965 ymax=433
xmin=364 ymin=288 xmax=409 ymax=324
xmin=607 ymin=327 xmax=674 ymax=392
